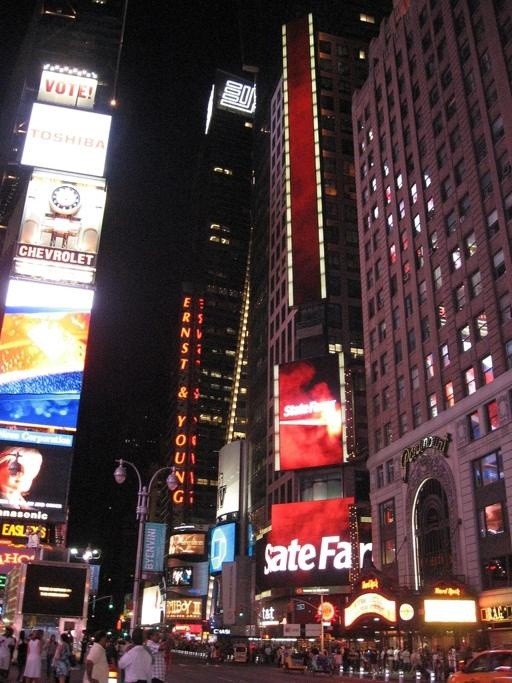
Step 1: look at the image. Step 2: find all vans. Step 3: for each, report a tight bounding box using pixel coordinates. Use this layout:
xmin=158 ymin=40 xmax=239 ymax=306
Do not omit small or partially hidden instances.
xmin=232 ymin=645 xmax=248 ymax=663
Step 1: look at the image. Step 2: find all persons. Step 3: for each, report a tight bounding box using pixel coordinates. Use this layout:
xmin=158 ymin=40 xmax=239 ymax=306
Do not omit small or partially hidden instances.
xmin=0 ymin=444 xmax=44 ymax=507
xmin=1 ymin=623 xmax=218 ymax=683
xmin=277 ymin=640 xmax=468 ymax=680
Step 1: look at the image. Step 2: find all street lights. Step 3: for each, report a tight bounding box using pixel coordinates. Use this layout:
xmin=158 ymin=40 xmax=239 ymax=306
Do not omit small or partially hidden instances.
xmin=0 ymin=450 xmax=26 ymax=482
xmin=239 ymin=611 xmax=252 ymax=638
xmin=112 ymin=456 xmax=178 ymax=652
xmin=69 ymin=543 xmax=102 ymax=565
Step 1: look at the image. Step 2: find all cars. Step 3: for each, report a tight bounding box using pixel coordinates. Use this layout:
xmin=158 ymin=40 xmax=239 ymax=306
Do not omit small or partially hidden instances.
xmin=447 ymin=648 xmax=512 ymax=683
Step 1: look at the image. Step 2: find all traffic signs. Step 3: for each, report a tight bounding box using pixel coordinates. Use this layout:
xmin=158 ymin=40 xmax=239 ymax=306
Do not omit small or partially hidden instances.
xmin=296 ymin=604 xmax=305 ymax=610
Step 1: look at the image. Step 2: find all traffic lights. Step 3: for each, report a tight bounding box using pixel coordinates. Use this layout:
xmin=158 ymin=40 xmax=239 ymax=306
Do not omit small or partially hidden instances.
xmin=108 ymin=599 xmax=114 ymax=611
